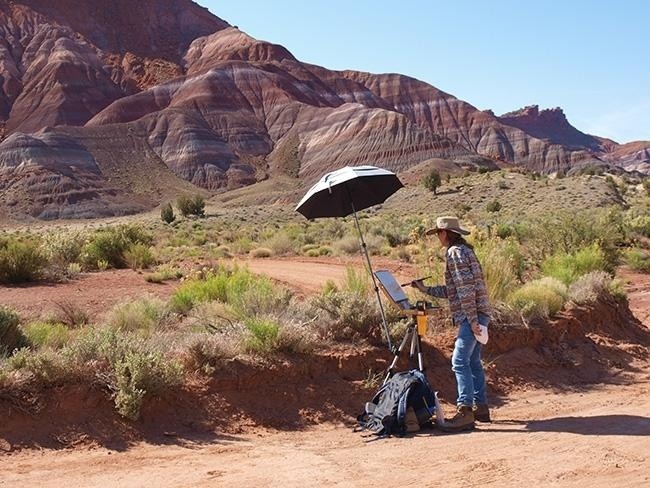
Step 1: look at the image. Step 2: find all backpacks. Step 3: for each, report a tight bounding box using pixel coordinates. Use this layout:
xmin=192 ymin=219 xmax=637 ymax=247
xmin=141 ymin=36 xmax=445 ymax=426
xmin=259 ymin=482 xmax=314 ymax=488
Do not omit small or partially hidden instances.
xmin=358 ymin=369 xmax=435 ymax=438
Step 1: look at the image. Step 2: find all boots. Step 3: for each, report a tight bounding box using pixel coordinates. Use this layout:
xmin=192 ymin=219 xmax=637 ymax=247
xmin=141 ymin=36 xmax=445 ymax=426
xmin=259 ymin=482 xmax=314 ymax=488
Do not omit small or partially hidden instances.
xmin=472 ymin=403 xmax=490 ymax=422
xmin=438 ymin=406 xmax=474 ymax=432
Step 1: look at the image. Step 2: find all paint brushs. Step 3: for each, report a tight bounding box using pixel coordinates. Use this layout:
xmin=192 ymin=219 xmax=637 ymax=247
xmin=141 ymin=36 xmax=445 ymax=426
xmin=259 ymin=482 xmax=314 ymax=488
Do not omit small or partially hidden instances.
xmin=400 ymin=276 xmax=432 ymax=288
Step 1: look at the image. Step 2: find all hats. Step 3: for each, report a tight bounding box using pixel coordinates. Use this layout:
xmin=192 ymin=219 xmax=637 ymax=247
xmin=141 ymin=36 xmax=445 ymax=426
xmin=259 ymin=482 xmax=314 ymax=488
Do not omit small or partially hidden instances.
xmin=426 ymin=217 xmax=471 ymax=236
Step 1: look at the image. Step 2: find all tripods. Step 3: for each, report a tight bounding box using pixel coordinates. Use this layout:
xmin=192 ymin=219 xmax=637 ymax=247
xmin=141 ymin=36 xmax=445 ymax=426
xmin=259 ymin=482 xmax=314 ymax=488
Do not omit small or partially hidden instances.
xmin=380 ymin=323 xmax=427 ymax=386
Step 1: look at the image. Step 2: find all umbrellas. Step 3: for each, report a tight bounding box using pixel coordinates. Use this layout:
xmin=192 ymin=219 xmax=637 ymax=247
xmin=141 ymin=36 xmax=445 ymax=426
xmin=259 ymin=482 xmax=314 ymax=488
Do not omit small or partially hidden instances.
xmin=293 ymin=164 xmax=406 ymax=351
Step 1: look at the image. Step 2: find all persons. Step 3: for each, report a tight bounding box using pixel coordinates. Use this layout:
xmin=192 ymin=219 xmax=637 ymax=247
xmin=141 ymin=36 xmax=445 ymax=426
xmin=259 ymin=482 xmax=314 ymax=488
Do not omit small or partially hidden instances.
xmin=412 ymin=215 xmax=492 ymax=431
